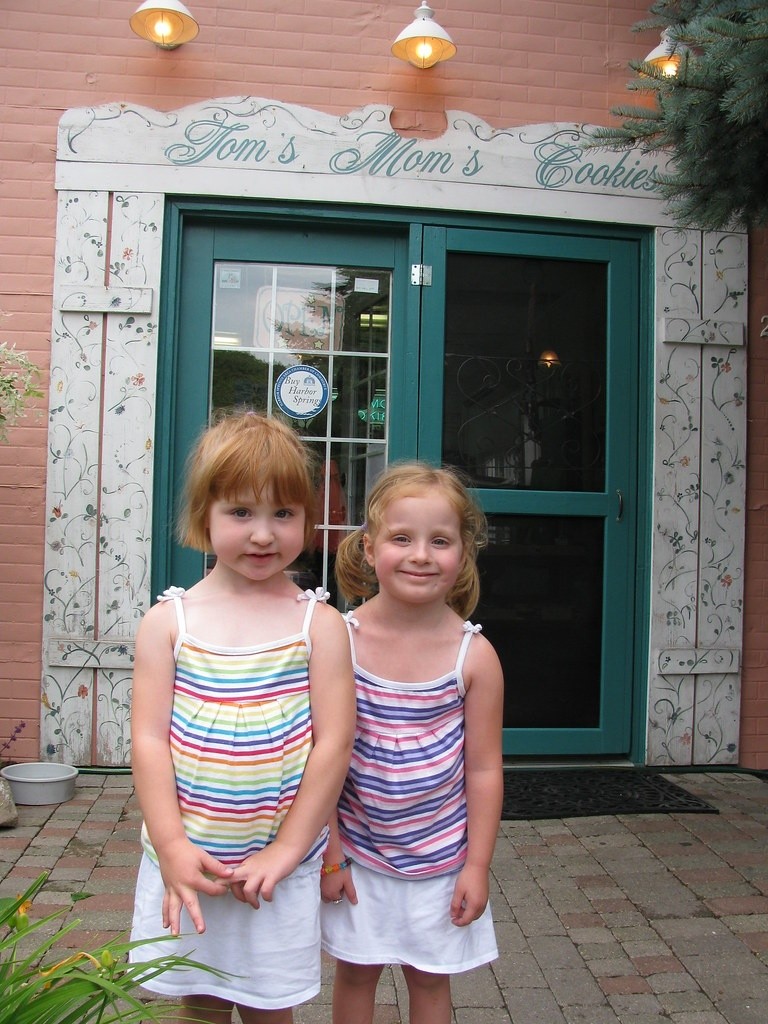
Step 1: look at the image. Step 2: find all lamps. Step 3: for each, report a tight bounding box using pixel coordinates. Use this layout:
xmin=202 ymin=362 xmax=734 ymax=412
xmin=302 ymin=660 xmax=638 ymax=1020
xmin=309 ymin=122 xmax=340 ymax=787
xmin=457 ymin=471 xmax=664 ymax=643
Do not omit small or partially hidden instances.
xmin=638 ymin=26 xmax=696 ymax=79
xmin=390 ymin=0 xmax=458 ymax=69
xmin=129 ymin=1 xmax=200 ymax=50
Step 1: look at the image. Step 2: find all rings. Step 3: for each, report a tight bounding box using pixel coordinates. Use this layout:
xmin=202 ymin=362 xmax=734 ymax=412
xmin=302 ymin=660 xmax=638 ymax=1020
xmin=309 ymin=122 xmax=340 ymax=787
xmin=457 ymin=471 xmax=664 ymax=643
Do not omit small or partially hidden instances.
xmin=333 ymin=899 xmax=343 ymax=905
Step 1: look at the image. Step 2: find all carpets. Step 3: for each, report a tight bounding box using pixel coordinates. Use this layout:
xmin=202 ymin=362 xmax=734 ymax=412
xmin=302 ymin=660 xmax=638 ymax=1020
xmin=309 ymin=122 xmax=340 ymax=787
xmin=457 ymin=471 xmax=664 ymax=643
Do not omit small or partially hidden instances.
xmin=501 ymin=770 xmax=719 ymax=820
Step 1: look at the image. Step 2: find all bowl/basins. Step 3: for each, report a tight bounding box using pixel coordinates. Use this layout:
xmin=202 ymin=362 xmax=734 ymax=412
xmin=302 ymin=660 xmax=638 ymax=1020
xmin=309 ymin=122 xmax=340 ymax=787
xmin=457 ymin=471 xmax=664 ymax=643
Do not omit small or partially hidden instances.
xmin=1 ymin=762 xmax=79 ymax=805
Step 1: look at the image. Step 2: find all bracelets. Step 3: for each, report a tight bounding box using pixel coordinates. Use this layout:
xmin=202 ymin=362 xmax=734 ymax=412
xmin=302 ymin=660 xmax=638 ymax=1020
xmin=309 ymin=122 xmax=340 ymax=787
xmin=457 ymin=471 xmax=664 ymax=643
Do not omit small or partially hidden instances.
xmin=321 ymin=857 xmax=354 ymax=875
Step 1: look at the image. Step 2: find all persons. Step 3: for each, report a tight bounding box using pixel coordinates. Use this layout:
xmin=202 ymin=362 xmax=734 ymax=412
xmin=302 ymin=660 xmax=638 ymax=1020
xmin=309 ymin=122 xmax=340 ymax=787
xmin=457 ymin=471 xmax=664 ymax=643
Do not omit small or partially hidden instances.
xmin=131 ymin=411 xmax=357 ymax=1024
xmin=319 ymin=466 xmax=498 ymax=1024
